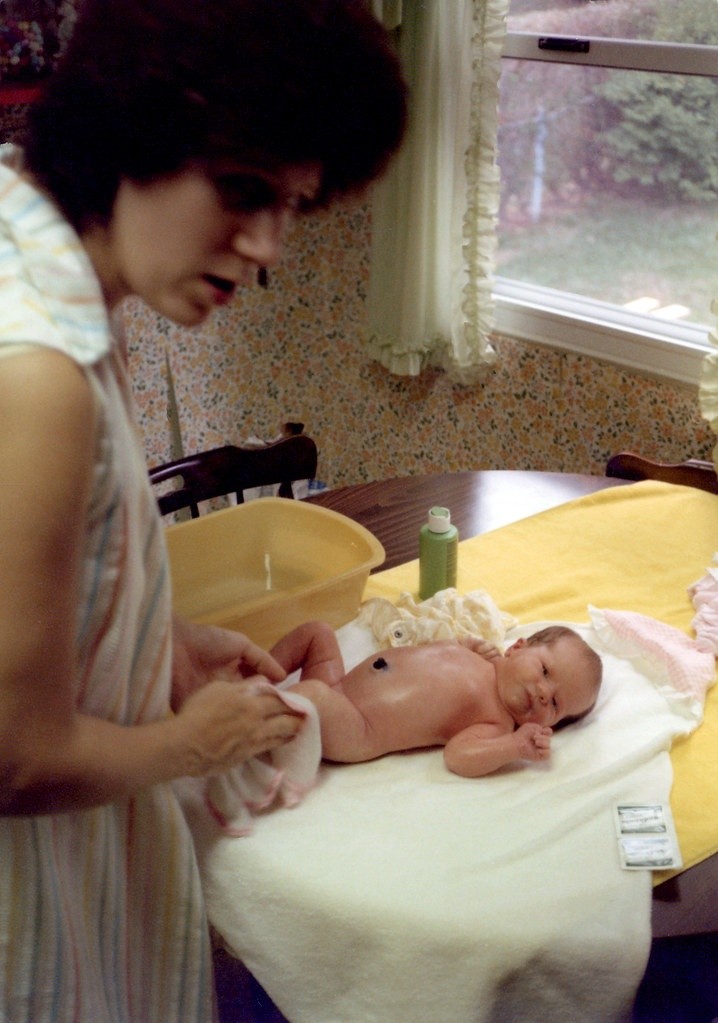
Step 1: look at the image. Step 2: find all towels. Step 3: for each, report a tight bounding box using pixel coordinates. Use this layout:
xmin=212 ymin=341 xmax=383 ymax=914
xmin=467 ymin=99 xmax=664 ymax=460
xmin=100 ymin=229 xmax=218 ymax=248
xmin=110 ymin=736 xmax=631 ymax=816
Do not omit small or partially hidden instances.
xmin=126 ymin=549 xmax=718 ymax=1023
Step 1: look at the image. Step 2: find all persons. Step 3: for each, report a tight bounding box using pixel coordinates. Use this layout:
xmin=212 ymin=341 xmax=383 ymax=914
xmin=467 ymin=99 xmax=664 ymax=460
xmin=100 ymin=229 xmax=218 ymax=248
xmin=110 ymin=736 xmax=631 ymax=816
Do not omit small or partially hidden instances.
xmin=0 ymin=0 xmax=410 ymax=1023
xmin=249 ymin=622 xmax=603 ymax=776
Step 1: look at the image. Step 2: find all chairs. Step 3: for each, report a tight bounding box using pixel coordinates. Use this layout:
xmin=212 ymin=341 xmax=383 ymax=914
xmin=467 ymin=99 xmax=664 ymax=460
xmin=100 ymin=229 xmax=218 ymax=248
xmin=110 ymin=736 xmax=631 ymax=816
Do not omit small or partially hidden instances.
xmin=605 ymin=453 xmax=718 ymax=495
xmin=147 ymin=421 xmax=319 ymax=519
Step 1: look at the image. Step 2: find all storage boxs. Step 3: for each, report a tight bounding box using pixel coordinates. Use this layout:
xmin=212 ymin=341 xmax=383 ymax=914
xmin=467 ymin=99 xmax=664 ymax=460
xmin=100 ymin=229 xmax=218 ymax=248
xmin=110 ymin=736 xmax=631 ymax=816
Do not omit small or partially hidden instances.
xmin=165 ymin=494 xmax=388 ymax=652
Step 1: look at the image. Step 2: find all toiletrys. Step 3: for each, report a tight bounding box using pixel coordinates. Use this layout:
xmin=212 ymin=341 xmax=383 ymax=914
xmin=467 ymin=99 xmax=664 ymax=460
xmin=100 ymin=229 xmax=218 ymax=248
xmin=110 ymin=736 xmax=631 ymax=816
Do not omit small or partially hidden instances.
xmin=418 ymin=505 xmax=458 ymax=603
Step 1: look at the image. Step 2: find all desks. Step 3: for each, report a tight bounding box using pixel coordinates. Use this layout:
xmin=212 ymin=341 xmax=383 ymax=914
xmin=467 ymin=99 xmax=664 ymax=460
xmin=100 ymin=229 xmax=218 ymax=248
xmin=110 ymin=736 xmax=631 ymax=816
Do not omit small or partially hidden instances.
xmin=213 ymin=468 xmax=716 ymax=996
